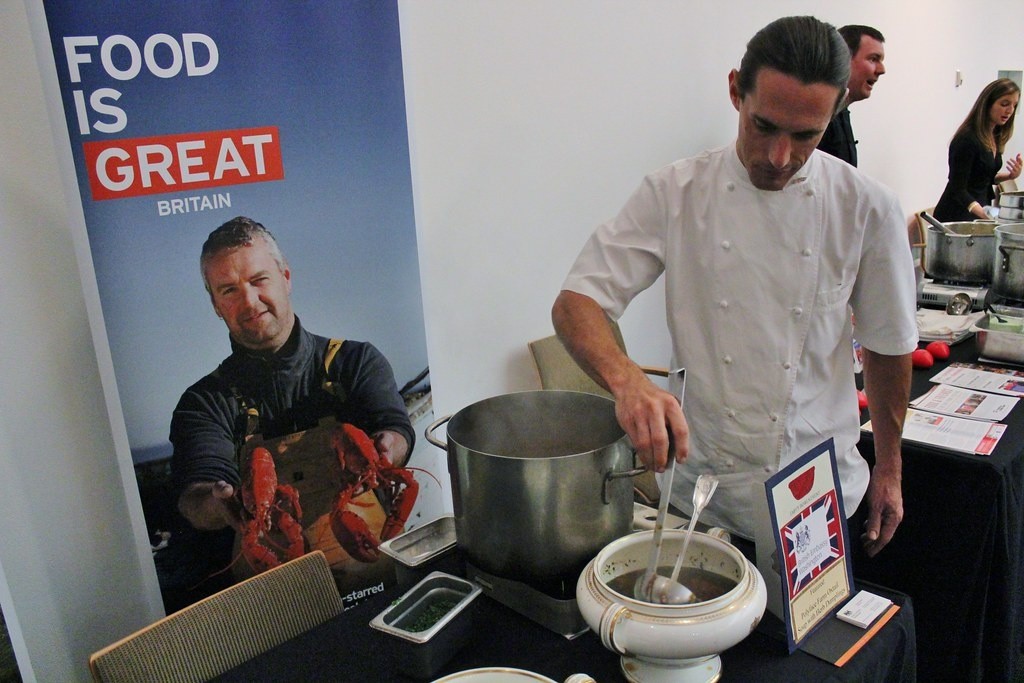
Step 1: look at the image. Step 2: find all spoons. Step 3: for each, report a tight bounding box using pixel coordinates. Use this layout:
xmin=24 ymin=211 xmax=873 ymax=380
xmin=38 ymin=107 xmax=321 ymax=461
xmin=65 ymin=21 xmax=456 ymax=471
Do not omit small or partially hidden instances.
xmin=985 ymin=301 xmax=1007 ymax=323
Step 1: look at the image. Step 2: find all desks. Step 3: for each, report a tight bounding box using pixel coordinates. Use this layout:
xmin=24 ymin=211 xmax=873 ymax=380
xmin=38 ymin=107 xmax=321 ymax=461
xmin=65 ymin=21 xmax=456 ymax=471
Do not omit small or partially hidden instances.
xmin=856 ymin=334 xmax=1024 ymax=683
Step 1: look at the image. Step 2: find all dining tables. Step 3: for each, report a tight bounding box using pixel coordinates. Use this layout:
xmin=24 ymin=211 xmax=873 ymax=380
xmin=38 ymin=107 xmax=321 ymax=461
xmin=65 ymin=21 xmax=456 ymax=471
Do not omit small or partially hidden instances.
xmin=204 ymin=507 xmax=916 ymax=683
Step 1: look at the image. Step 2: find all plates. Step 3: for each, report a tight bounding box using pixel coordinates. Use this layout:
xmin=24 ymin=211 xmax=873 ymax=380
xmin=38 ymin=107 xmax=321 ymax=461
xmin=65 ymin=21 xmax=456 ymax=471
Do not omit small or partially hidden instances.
xmin=429 ymin=667 xmax=558 ymax=683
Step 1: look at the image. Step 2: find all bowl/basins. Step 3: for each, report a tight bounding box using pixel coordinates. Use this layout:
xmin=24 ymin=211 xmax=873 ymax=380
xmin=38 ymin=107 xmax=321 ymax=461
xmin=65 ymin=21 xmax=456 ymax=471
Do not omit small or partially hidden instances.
xmin=576 ymin=528 xmax=767 ymax=683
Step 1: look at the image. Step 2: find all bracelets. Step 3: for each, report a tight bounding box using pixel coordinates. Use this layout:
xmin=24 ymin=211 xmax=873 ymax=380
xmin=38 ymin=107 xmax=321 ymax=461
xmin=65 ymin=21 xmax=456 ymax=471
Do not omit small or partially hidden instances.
xmin=982 ymin=212 xmax=987 ymax=219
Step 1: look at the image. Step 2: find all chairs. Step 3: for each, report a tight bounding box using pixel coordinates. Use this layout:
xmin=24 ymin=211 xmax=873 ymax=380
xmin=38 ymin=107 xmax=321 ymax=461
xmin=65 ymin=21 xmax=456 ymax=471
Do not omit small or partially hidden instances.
xmin=912 ymin=208 xmax=933 ymax=272
xmin=88 ymin=550 xmax=344 ymax=683
xmin=527 ymin=323 xmax=669 ymax=506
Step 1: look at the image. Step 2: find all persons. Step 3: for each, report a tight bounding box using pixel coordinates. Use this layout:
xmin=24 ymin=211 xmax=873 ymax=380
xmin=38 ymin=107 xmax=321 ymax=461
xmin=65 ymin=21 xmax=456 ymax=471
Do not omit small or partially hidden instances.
xmin=169 ymin=216 xmax=415 ymax=585
xmin=958 ymin=396 xmax=984 ymax=414
xmin=933 ymin=78 xmax=1024 ymax=222
xmin=1003 ymin=380 xmax=1018 ymax=390
xmin=815 ymin=24 xmax=886 ymax=170
xmin=552 ymin=15 xmax=919 ymax=561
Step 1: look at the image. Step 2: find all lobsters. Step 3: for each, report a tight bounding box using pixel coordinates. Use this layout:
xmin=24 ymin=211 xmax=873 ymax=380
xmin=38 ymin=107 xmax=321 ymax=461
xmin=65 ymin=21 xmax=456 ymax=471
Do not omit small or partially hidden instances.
xmin=325 ymin=422 xmax=420 ymax=564
xmin=237 ymin=445 xmax=305 ymax=575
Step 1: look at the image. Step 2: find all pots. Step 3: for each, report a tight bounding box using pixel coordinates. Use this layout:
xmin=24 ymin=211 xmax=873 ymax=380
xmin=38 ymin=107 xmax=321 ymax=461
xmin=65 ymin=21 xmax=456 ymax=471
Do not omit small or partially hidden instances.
xmin=924 ymin=219 xmax=1003 ymax=282
xmin=424 ymin=390 xmax=649 ymax=582
xmin=991 ymin=222 xmax=1024 ymax=299
xmin=998 ymin=191 xmax=1024 ymax=222
